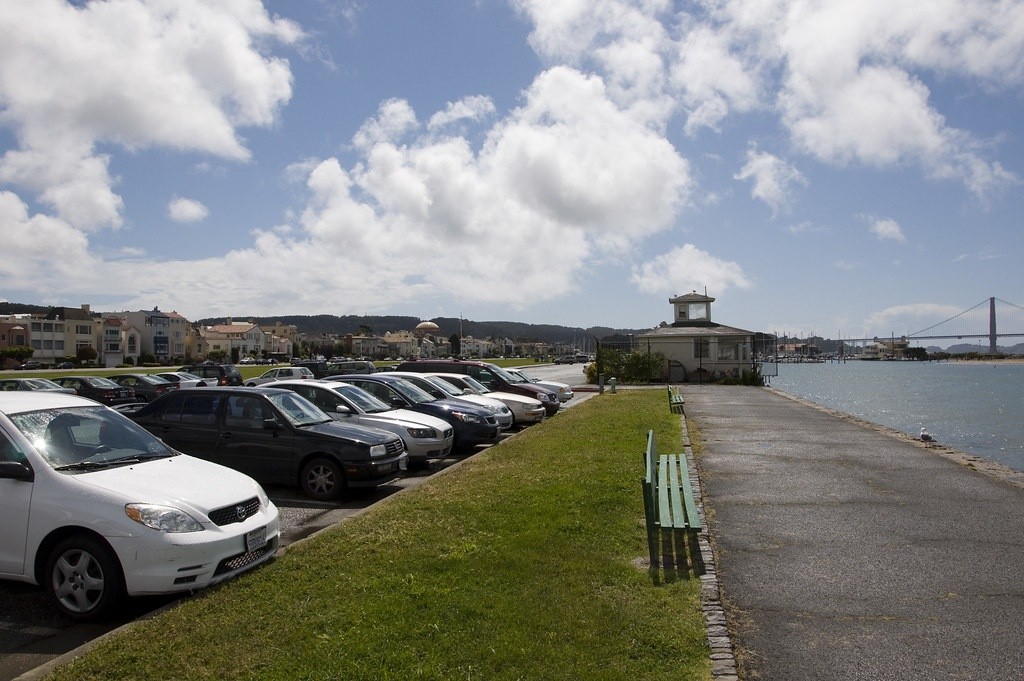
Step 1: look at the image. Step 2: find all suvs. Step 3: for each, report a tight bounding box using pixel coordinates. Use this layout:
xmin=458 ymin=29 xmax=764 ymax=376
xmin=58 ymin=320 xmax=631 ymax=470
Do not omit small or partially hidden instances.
xmin=554 ymin=356 xmax=577 ymax=365
xmin=576 ymin=355 xmax=590 ymax=364
xmin=394 ymin=357 xmax=561 ymax=415
xmin=240 ymin=357 xmax=255 ymax=365
xmin=175 ymin=361 xmax=245 ymax=388
xmin=19 ymin=361 xmax=40 ymax=370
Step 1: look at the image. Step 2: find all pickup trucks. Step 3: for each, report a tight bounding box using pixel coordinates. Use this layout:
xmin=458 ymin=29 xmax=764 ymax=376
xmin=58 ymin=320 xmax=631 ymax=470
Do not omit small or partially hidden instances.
xmin=292 ymin=361 xmax=357 ymax=377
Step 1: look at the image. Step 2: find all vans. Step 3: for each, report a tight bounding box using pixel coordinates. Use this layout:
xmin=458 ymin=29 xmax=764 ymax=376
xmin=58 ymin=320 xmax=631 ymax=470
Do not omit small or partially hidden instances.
xmin=327 ymin=361 xmax=377 ymax=376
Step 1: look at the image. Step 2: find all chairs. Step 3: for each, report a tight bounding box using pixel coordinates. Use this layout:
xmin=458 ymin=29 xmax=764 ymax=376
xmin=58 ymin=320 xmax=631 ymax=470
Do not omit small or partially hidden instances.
xmin=243 ymin=405 xmax=257 ymax=420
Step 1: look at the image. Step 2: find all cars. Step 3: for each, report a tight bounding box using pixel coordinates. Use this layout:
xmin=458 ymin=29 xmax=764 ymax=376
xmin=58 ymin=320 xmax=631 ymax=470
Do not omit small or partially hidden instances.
xmin=50 ymin=376 xmax=137 ymax=405
xmin=224 ymin=379 xmax=454 ymax=468
xmin=109 ymin=373 xmax=175 ymax=402
xmin=154 ymin=372 xmax=219 ymax=389
xmin=0 ymin=390 xmax=281 ymax=618
xmin=256 ymin=359 xmax=270 ymax=364
xmin=478 ymin=369 xmax=575 ymax=403
xmin=243 ymin=367 xmax=315 ymax=389
xmin=362 ymin=372 xmax=513 ymax=430
xmin=269 ymin=358 xmax=279 ymax=365
xmin=101 ymin=385 xmax=410 ymax=504
xmin=288 ymin=355 xmax=406 ymax=362
xmin=425 ymin=374 xmax=546 ymax=426
xmin=55 ymin=362 xmax=75 ymax=369
xmin=321 ymin=375 xmax=501 ymax=450
xmin=0 ymin=378 xmax=77 ymax=397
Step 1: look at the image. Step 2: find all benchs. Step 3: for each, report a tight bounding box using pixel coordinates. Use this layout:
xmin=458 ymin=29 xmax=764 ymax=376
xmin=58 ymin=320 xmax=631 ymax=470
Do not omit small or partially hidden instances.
xmin=667 ymin=385 xmax=685 ymax=413
xmin=641 ymin=429 xmax=702 ymax=565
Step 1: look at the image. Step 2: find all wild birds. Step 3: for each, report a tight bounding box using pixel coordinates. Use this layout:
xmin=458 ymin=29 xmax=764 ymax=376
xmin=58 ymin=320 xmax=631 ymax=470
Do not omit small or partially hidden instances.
xmin=920 ymin=427 xmax=938 ymax=447
